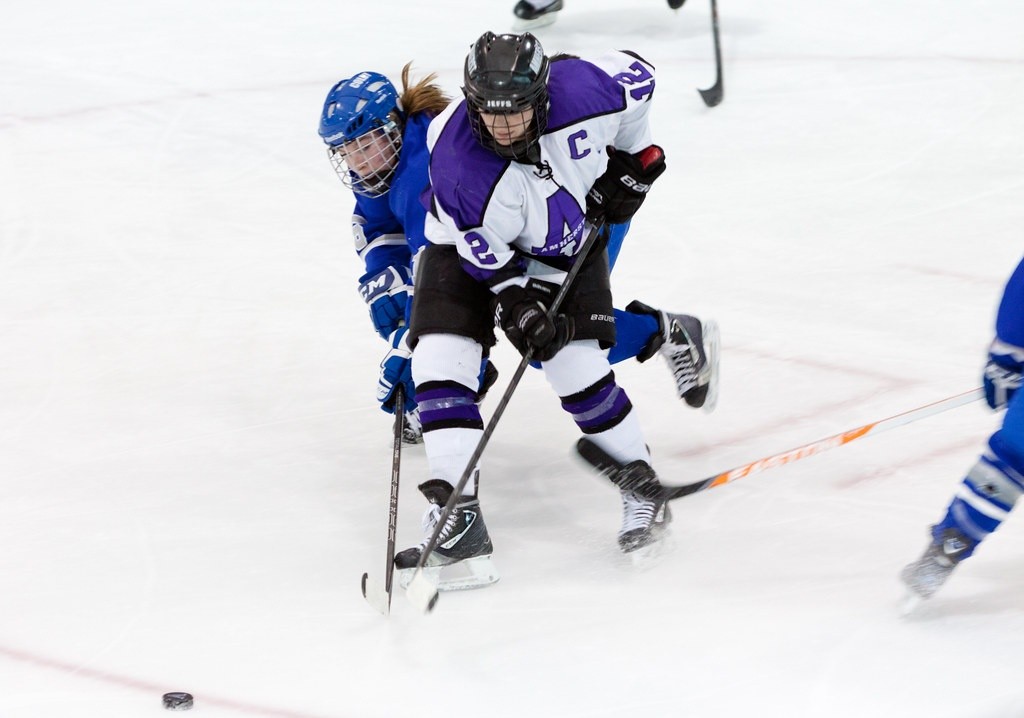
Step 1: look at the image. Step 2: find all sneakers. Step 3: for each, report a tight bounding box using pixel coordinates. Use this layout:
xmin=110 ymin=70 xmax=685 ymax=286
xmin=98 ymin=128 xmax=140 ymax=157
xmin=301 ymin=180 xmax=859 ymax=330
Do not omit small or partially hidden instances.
xmin=666 ymin=0 xmax=684 ymax=10
xmin=394 ymin=468 xmax=501 ymax=592
xmin=511 ymin=0 xmax=564 ymax=29
xmin=617 ymin=442 xmax=675 ymax=557
xmin=898 ymin=525 xmax=974 ymax=617
xmin=625 ymin=298 xmax=723 ymax=415
xmin=391 ymin=360 xmax=499 ymax=445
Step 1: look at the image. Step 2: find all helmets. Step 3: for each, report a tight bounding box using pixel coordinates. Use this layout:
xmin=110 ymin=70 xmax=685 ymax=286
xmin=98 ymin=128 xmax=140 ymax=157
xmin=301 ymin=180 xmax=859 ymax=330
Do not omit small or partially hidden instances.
xmin=459 ymin=30 xmax=552 ymax=154
xmin=318 ymin=69 xmax=406 ymax=200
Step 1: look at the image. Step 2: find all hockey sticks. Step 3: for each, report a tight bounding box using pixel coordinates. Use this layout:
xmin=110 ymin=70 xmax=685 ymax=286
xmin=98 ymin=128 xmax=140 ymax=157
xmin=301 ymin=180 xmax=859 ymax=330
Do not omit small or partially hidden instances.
xmin=359 ymin=207 xmax=627 ymax=611
xmin=696 ymin=0 xmax=723 ymax=107
xmin=382 ymin=199 xmax=461 ymax=615
xmin=576 ymin=390 xmax=986 ymax=502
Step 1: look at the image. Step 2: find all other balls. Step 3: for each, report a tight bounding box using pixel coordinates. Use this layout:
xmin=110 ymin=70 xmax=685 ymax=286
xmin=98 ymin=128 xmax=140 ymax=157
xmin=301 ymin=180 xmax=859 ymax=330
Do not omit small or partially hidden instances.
xmin=162 ymin=691 xmax=194 ymax=710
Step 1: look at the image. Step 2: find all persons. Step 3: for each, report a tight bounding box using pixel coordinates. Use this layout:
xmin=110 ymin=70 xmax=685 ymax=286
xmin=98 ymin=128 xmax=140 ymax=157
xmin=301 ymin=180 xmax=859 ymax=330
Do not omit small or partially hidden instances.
xmin=894 ymin=251 xmax=1024 ymax=617
xmin=393 ymin=32 xmax=675 ymax=596
xmin=316 ymin=71 xmax=720 ymax=449
